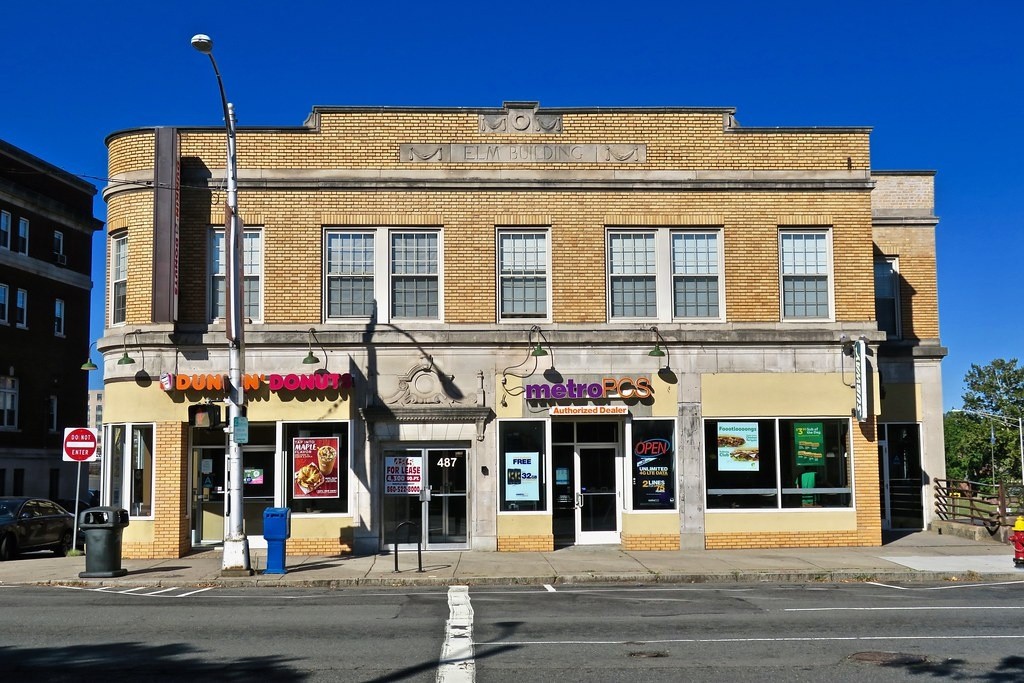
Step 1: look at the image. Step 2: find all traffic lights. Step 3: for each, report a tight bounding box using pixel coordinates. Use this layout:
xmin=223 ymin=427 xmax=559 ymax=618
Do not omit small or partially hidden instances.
xmin=189 ymin=403 xmax=221 ymax=429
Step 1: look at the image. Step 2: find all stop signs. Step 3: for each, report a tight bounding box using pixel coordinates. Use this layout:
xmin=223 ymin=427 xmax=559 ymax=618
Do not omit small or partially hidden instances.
xmin=62 ymin=427 xmax=98 ymax=462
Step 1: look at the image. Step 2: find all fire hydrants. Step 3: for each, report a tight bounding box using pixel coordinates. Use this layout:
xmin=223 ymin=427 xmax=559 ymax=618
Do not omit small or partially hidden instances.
xmin=1009 ymin=515 xmax=1024 ymax=568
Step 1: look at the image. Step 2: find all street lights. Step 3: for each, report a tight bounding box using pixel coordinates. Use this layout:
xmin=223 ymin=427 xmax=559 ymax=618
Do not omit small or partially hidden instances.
xmin=951 ymin=408 xmax=1024 ymax=483
xmin=191 ymin=33 xmax=255 ymax=578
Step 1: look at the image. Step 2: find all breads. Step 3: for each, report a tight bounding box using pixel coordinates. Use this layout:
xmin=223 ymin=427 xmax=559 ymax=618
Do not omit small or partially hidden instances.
xmin=296 ymin=465 xmax=322 ymax=490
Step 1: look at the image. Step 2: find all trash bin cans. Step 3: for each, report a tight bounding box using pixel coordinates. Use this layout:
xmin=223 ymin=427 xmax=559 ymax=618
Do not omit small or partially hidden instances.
xmin=78 ymin=506 xmax=129 ymax=578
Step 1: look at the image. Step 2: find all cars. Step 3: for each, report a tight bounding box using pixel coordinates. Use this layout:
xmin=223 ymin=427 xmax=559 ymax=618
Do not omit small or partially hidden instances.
xmin=0 ymin=496 xmax=75 ymax=560
xmin=57 ymin=497 xmax=91 ymax=515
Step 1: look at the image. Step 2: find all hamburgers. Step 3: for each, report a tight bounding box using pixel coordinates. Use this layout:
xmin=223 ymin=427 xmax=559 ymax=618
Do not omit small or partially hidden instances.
xmin=718 ymin=434 xmax=746 ymax=447
xmin=730 ymin=447 xmax=759 ymax=461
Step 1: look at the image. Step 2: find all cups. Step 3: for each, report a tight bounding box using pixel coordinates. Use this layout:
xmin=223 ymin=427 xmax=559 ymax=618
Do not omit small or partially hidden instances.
xmin=318 ymin=446 xmax=336 ymax=476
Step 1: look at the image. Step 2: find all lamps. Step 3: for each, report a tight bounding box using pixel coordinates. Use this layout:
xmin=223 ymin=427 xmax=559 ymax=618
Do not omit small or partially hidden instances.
xmin=530 ymin=327 xmax=547 ymax=356
xmin=80 ymin=341 xmax=98 ymax=370
xmin=117 ymin=330 xmax=140 ymax=364
xmin=648 ymin=326 xmax=665 ymax=357
xmin=303 ymin=328 xmax=319 ymax=364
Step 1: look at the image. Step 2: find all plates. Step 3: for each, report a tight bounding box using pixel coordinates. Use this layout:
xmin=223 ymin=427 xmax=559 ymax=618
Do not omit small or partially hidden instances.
xmin=295 ymin=462 xmax=325 ymax=495
